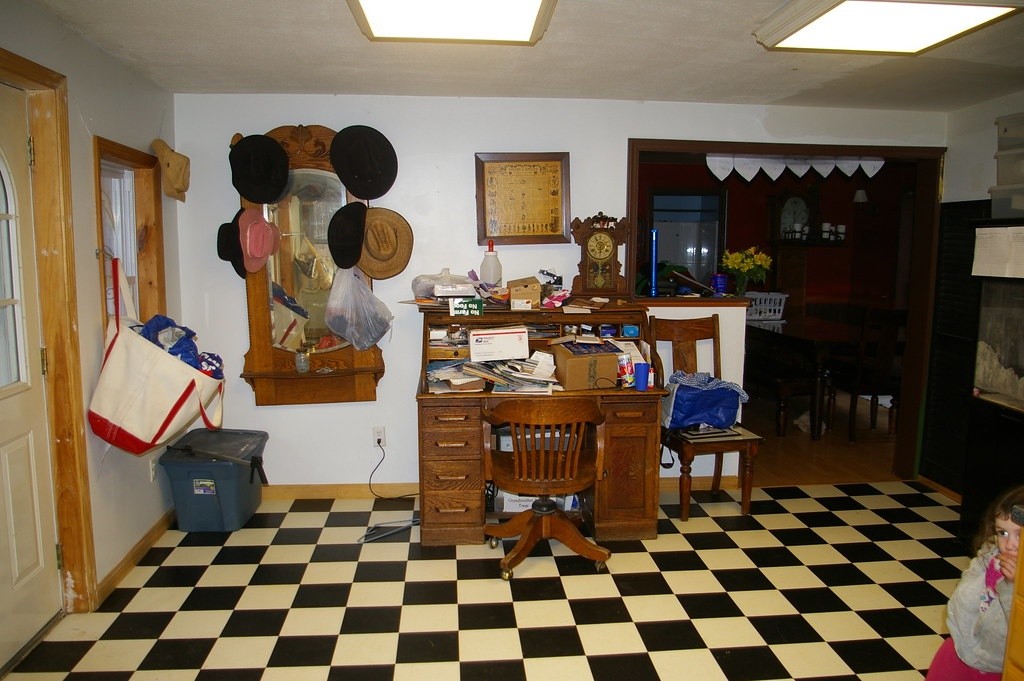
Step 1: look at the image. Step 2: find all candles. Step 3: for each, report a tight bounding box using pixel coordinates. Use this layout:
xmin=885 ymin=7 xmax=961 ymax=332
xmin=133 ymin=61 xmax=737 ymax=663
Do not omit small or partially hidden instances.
xmin=830 ymin=227 xmax=836 ymax=240
xmin=793 ymin=223 xmax=802 ymax=238
xmin=822 ymin=223 xmax=831 ymax=238
xmin=837 ymin=225 xmax=846 ymax=239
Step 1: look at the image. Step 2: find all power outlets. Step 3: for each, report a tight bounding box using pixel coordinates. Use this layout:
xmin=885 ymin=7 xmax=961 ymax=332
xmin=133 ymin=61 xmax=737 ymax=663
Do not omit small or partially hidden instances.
xmin=372 ymin=425 xmax=386 ymax=447
xmin=149 ymin=455 xmax=158 ymax=482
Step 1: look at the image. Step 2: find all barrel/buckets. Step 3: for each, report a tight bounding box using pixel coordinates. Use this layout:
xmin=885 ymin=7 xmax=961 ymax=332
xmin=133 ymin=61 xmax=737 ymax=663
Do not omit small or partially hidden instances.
xmin=710 ymin=274 xmax=728 ymax=293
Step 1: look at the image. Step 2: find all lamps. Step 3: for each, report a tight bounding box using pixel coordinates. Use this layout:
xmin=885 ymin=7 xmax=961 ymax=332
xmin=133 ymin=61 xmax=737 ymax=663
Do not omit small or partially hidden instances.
xmin=853 ymin=188 xmax=868 ymax=214
xmin=345 ymin=0 xmax=558 ymax=47
xmin=752 ymin=0 xmax=1024 ymax=58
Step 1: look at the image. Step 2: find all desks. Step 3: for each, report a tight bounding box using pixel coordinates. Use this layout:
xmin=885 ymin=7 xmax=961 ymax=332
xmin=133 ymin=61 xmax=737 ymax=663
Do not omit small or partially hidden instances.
xmin=750 ymin=314 xmax=907 ymax=440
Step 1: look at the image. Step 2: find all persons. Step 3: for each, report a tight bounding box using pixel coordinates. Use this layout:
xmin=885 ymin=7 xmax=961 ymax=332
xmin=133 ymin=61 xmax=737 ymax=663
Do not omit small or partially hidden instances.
xmin=926 ymin=486 xmax=1024 ymax=681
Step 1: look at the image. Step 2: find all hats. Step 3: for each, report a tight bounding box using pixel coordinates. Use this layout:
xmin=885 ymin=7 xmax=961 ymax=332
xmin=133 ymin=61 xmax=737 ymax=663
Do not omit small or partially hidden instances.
xmin=268 ymin=221 xmax=281 ymax=255
xmin=238 ymin=207 xmax=273 ymax=273
xmin=228 ymin=135 xmax=289 ymax=204
xmin=326 ymin=201 xmax=368 ymax=270
xmin=356 ymin=207 xmax=413 ymax=280
xmin=152 ymin=139 xmax=190 ymax=203
xmin=216 ymin=207 xmax=246 ymax=279
xmin=330 ymin=125 xmax=398 ymax=200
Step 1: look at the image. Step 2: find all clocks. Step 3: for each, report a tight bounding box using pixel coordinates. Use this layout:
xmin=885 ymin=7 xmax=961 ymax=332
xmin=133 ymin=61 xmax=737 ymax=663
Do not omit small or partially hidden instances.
xmin=570 ymin=210 xmax=632 ymax=302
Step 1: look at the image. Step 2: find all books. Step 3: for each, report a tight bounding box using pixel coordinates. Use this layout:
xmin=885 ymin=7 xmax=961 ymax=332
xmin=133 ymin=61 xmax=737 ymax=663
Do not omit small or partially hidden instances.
xmin=548 ymin=336 xmax=605 ymax=345
xmin=460 ymin=360 xmax=559 ymax=396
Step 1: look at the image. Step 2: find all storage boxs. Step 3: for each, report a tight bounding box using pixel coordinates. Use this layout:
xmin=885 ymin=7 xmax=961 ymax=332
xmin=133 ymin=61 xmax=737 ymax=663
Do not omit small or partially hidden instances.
xmin=466 ymin=324 xmax=529 ymax=362
xmin=550 ymin=343 xmax=618 ymax=391
xmin=159 ymin=427 xmax=270 ymax=533
xmin=744 ymin=291 xmax=790 ymax=319
xmin=507 ymin=276 xmax=543 ymax=309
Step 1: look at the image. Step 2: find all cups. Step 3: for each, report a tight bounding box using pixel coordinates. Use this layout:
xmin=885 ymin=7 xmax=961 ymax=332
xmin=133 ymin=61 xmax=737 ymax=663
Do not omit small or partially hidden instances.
xmin=822 ymin=223 xmax=846 ymax=241
xmin=782 ymin=224 xmax=809 ymax=240
xmin=635 ymin=363 xmax=650 ymax=391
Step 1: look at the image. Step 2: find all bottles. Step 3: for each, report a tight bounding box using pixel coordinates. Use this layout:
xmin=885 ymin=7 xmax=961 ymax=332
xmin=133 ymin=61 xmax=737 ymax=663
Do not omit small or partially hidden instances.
xmin=480 ymin=240 xmax=502 ymax=289
xmin=302 ymin=343 xmax=315 ymax=353
xmin=295 ymin=349 xmax=310 ymax=373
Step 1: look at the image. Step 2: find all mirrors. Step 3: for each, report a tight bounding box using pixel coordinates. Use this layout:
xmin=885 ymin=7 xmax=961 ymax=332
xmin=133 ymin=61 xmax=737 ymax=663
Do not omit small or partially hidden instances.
xmin=229 ymin=124 xmax=386 ymax=406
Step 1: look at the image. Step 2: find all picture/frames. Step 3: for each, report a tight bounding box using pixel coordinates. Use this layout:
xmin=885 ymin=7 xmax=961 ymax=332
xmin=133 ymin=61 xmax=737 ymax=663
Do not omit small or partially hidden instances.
xmin=474 ymin=152 xmax=572 ymax=246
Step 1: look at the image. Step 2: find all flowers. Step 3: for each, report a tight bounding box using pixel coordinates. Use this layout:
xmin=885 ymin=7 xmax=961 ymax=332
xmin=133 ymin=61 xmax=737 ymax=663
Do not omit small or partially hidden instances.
xmin=722 ymin=245 xmax=773 ymax=296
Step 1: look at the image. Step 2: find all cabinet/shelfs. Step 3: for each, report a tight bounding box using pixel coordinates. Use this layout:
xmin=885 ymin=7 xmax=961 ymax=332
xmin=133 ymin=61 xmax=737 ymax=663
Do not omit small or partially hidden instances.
xmin=414 ymin=304 xmax=670 ymax=548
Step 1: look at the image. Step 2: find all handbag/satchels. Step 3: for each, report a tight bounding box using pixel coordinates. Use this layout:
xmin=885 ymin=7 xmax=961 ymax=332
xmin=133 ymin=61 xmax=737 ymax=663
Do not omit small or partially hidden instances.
xmin=295 ymin=236 xmax=334 ymax=293
xmin=325 ymin=264 xmax=394 ymax=352
xmin=88 ymin=258 xmax=226 ymax=456
xmin=273 ymin=283 xmax=309 ymax=350
xmin=658 ymin=378 xmax=739 ymax=469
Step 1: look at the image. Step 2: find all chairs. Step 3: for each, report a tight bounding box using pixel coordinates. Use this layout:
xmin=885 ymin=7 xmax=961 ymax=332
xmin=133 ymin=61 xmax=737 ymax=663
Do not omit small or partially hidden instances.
xmin=650 ymin=314 xmax=764 ymax=521
xmin=826 ymin=303 xmax=911 ymax=444
xmin=483 ymin=396 xmax=611 ymax=580
xmin=746 ymin=326 xmax=836 ymax=437
